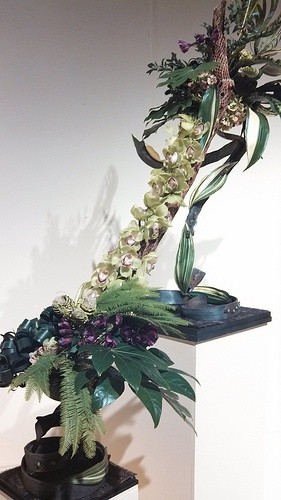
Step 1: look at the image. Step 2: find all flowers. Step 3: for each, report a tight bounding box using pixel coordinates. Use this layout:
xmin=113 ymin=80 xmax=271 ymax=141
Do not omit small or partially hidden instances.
xmin=0 ymin=1 xmax=279 ymax=460
